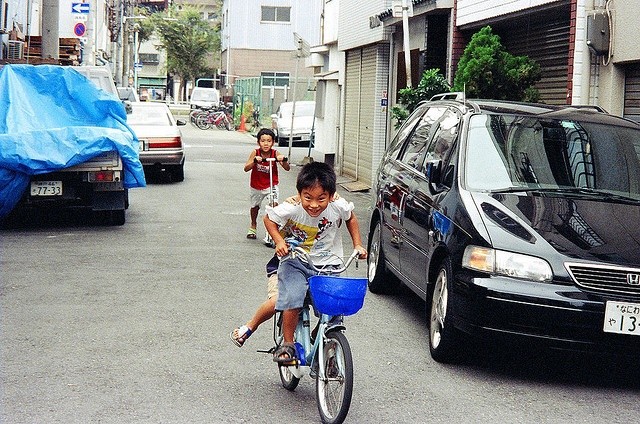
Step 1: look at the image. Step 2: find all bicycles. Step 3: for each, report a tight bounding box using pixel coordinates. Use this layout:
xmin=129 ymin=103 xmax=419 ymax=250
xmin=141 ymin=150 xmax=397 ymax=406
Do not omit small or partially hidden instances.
xmin=189 ymin=106 xmax=237 ymax=130
xmin=256 ymin=235 xmax=368 ymax=424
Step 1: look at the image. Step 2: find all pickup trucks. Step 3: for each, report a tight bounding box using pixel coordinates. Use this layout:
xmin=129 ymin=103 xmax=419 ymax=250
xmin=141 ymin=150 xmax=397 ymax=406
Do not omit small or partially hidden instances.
xmin=0 ymin=64 xmax=129 ymax=230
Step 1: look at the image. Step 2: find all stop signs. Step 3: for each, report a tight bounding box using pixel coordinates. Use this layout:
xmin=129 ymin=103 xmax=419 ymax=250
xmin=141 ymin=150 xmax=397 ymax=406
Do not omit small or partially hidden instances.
xmin=73 ymin=21 xmax=86 ymax=37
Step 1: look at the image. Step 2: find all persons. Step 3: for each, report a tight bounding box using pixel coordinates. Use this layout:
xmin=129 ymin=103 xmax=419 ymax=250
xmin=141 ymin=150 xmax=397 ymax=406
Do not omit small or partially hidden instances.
xmin=244 ymin=128 xmax=290 ymax=239
xmin=264 ymin=161 xmax=368 ymax=362
xmin=229 ymin=192 xmax=341 ymax=348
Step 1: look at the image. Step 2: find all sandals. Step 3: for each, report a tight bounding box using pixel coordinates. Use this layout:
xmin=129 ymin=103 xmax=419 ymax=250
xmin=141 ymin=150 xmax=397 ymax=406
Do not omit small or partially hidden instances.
xmin=230 ymin=325 xmax=252 ymax=347
xmin=247 ymin=228 xmax=257 ymax=238
xmin=273 ymin=342 xmax=296 ymax=361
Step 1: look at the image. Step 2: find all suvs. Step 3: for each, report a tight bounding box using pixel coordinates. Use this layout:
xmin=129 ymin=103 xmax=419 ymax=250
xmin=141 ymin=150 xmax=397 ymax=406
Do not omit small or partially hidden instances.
xmin=367 ymin=91 xmax=640 ymax=365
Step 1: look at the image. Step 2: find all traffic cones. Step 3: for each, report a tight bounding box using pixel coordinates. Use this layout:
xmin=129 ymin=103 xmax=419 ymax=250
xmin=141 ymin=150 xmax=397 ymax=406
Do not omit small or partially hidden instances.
xmin=237 ymin=112 xmax=248 ymax=132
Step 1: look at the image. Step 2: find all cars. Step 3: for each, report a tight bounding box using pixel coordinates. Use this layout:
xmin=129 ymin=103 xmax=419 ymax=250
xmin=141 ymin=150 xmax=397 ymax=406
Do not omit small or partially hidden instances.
xmin=271 ymin=101 xmax=316 ymax=147
xmin=123 ymin=101 xmax=186 ymax=183
xmin=117 ymin=85 xmax=141 ymax=104
xmin=190 ymin=86 xmax=222 ymax=110
